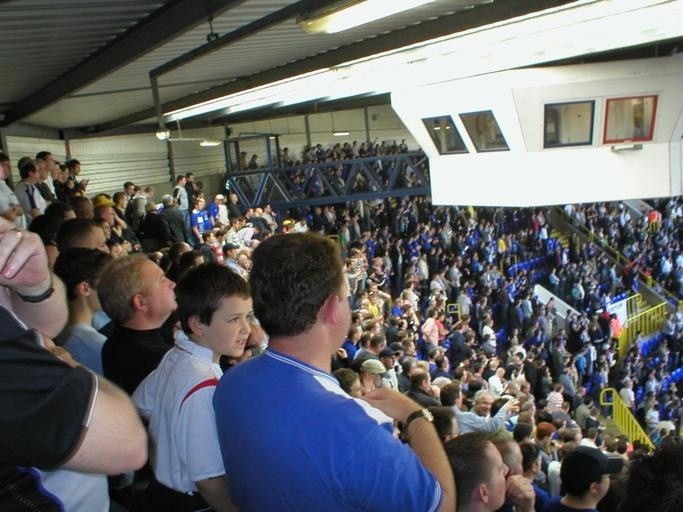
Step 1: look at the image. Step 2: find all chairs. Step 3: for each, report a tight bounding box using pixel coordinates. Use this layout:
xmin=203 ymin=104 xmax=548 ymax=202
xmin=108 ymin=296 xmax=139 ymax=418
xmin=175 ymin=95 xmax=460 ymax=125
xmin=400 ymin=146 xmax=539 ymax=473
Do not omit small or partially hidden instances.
xmin=642 ymin=334 xmax=664 ymax=358
xmin=508 ymin=256 xmax=545 ymax=282
xmin=659 ymin=368 xmax=683 ymax=395
xmin=613 ymin=293 xmax=627 ymax=302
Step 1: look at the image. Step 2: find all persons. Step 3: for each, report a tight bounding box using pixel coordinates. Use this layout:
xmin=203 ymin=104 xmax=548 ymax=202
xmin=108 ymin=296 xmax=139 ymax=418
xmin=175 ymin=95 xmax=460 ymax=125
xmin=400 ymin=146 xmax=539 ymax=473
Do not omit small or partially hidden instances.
xmin=1 ymin=136 xmax=682 ymax=510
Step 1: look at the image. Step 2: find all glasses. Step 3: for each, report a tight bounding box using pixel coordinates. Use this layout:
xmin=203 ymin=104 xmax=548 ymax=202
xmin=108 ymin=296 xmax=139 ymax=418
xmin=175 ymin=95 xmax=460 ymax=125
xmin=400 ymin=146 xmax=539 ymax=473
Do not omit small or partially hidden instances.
xmin=89 ymin=248 xmax=104 ymax=267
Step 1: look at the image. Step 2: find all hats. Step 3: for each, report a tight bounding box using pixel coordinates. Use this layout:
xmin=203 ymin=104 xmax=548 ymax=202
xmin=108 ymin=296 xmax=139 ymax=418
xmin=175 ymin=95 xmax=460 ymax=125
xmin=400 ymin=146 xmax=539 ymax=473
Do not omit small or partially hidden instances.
xmin=93 ymin=195 xmax=115 ymax=208
xmin=222 ymin=243 xmax=240 ymax=251
xmin=216 ymin=194 xmax=223 ymax=199
xmin=519 ymin=442 xmax=539 ymax=471
xmin=359 ymin=358 xmax=390 ymax=378
xmin=560 ymin=446 xmax=623 ymax=482
xmin=389 ymin=342 xmax=406 ymax=351
xmin=380 ymin=347 xmax=399 ymax=357
xmin=434 ymin=346 xmax=446 ymax=353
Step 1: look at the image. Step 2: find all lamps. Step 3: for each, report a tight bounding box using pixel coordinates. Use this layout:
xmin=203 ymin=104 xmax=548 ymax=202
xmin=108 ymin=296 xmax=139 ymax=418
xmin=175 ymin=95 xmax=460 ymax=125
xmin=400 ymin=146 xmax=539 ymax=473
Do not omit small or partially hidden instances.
xmin=332 ymin=132 xmax=351 ymax=136
xmin=295 ymin=0 xmax=435 ymax=35
xmin=155 ymin=115 xmax=170 ymax=141
xmin=200 ymin=141 xmax=221 ymax=146
